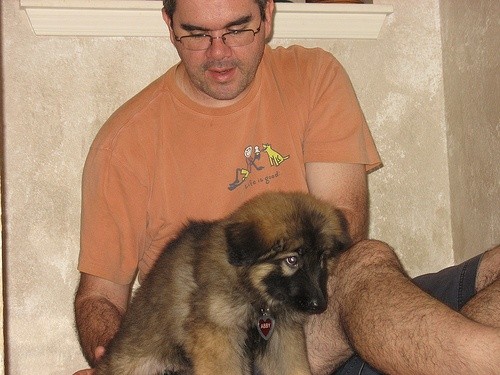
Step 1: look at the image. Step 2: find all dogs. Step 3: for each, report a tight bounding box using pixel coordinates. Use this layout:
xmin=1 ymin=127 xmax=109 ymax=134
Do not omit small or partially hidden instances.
xmin=91 ymin=189 xmax=353 ymax=375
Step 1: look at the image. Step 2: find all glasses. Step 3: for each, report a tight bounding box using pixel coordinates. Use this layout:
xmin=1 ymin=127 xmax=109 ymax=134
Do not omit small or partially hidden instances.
xmin=170 ymin=4 xmax=264 ymax=51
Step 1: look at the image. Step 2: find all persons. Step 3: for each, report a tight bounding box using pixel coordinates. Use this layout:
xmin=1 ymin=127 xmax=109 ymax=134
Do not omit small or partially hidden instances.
xmin=70 ymin=0 xmax=500 ymax=374
